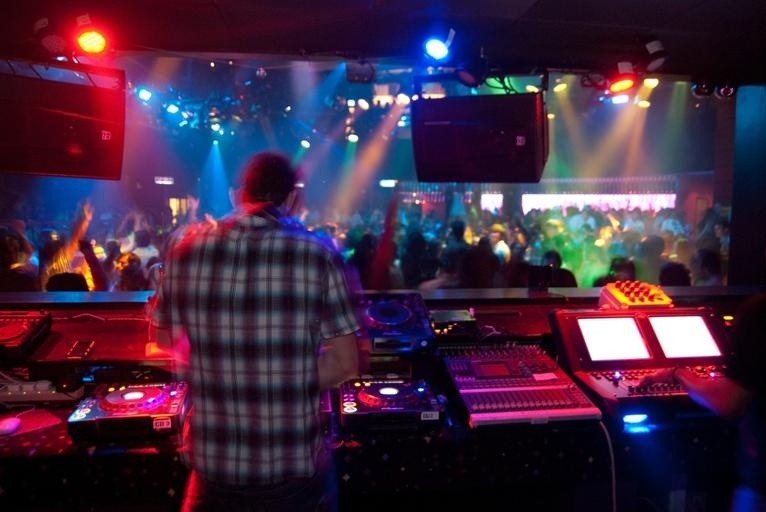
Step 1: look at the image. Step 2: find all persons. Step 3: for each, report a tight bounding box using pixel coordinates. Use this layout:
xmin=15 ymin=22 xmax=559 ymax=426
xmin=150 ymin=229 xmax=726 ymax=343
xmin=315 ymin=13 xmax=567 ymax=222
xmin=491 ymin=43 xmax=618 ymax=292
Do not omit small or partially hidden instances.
xmin=142 ymin=152 xmax=372 ymax=512
xmin=630 ymin=294 xmax=766 ymax=511
xmin=0 ymin=179 xmax=730 ymax=294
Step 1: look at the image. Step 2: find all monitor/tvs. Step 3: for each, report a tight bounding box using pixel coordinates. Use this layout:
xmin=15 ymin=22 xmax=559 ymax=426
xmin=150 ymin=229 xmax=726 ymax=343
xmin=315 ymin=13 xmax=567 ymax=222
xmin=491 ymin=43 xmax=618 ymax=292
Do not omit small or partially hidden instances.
xmin=648 ymin=315 xmax=723 ymax=361
xmin=577 ymin=314 xmax=653 ymax=363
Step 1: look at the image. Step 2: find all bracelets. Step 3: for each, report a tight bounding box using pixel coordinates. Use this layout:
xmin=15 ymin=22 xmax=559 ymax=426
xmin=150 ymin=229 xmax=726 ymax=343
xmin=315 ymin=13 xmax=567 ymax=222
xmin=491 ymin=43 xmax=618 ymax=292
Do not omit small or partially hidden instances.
xmin=671 ymin=366 xmax=687 ymax=385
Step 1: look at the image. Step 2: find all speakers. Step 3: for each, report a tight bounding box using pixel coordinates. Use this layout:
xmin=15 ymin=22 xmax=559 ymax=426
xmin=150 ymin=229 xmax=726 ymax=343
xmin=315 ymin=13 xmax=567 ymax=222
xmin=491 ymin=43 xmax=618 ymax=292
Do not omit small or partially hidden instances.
xmin=411 ymin=92 xmax=549 ymax=182
xmin=0 ymin=60 xmax=126 ymax=181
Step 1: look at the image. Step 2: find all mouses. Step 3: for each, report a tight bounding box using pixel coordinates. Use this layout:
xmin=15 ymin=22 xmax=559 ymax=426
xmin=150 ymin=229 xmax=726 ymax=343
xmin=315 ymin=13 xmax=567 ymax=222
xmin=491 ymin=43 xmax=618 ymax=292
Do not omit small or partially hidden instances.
xmin=0 ymin=417 xmax=21 ymax=434
